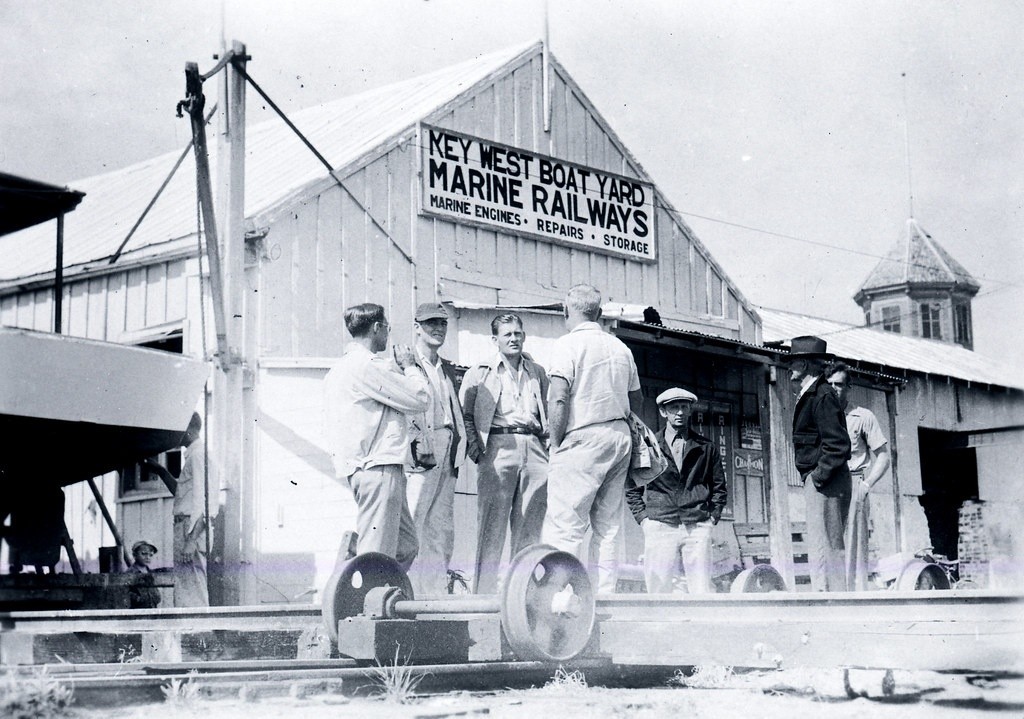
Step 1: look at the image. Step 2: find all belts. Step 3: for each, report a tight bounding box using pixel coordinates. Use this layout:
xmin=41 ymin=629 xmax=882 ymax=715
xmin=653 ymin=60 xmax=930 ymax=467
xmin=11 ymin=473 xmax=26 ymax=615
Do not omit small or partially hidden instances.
xmin=490 ymin=427 xmax=538 ymax=436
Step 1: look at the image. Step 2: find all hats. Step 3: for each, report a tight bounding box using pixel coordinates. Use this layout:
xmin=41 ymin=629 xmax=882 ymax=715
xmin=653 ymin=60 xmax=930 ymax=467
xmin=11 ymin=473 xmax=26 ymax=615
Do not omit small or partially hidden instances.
xmin=415 ymin=303 xmax=448 ymax=323
xmin=656 ymin=387 xmax=698 ymax=406
xmin=132 ymin=541 xmax=157 ymax=554
xmin=783 ymin=336 xmax=836 ymax=361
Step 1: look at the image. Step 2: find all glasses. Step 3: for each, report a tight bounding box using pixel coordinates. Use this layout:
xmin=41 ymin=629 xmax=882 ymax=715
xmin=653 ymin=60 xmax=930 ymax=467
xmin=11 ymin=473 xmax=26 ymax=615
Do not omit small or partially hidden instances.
xmin=373 ymin=320 xmax=392 ymax=333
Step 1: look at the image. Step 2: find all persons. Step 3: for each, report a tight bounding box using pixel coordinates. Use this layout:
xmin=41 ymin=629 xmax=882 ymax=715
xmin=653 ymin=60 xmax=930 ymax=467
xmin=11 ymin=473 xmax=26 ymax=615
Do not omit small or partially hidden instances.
xmin=407 ymin=303 xmax=467 ymax=598
xmin=625 ymin=388 xmax=727 ymax=593
xmin=124 ymin=540 xmax=161 ymax=609
xmin=825 ymin=362 xmax=890 ymax=591
xmin=323 ymin=303 xmax=430 ymax=588
xmin=782 ymin=336 xmax=851 ymax=592
xmin=457 ymin=313 xmax=548 ymax=594
xmin=545 ymin=284 xmax=642 ymax=594
